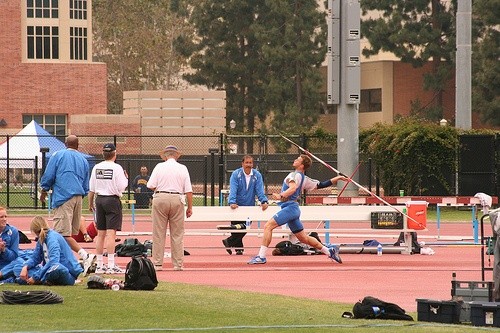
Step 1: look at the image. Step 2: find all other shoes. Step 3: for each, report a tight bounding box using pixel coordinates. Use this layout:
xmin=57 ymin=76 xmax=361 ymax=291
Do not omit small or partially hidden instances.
xmin=222 ymin=239 xmax=243 ymax=255
xmin=83 ymin=233 xmax=93 ymax=243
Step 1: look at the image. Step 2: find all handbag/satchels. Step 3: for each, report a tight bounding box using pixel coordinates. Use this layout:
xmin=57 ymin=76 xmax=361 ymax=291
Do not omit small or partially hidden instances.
xmin=271 ymin=240 xmax=305 ymax=256
xmin=352 ymin=295 xmax=414 ymax=321
xmin=117 ymin=237 xmax=149 ymax=258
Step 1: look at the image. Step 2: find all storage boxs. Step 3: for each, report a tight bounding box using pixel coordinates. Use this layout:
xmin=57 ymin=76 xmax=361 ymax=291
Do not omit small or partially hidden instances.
xmin=412 ymin=278 xmax=500 ymax=327
xmin=114 ymin=236 xmax=158 ymax=291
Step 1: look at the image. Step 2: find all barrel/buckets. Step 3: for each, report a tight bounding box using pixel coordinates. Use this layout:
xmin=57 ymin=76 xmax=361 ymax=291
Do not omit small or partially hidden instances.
xmin=405 ymin=200 xmax=429 ymax=230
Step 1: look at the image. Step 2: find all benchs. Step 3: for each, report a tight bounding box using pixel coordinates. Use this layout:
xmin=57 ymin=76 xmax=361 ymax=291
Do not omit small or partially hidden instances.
xmin=111 ymin=203 xmax=416 ymax=256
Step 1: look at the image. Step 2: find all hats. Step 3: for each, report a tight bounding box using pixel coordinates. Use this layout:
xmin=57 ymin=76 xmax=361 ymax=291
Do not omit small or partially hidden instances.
xmin=102 ymin=143 xmax=116 ymax=152
xmin=159 ymin=145 xmax=183 ymax=160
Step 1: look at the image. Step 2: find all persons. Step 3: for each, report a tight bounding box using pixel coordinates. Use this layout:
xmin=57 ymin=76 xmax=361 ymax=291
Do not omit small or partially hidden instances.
xmin=147 ymin=145 xmax=193 ymax=272
xmin=132 ymin=165 xmax=153 ymax=209
xmin=13 ymin=216 xmax=84 ymax=286
xmin=88 ymin=143 xmax=128 ymax=274
xmin=0 ymin=208 xmax=19 ymax=280
xmin=248 ymin=153 xmax=349 ymax=265
xmin=221 ymin=154 xmax=269 ymax=255
xmin=42 ymin=135 xmax=97 ymax=277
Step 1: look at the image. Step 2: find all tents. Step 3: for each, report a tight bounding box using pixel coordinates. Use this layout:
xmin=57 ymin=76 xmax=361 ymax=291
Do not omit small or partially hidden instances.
xmin=0 ymin=119 xmax=94 ymax=172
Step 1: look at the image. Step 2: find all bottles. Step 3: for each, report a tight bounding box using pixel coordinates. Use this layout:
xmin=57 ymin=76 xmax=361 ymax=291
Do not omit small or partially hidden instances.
xmin=377 ymin=243 xmax=383 ymax=256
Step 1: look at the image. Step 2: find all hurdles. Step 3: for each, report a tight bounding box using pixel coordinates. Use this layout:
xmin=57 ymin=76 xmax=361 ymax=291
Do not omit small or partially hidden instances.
xmin=436 ymin=202 xmax=476 ymax=241
xmin=119 ymin=199 xmax=136 ymax=235
xmin=47 ymin=189 xmax=53 ymax=221
xmin=257 ymin=220 xmax=288 ymax=238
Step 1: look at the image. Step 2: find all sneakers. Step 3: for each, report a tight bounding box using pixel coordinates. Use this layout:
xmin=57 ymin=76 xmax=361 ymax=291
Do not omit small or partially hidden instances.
xmin=327 ymin=247 xmax=343 ymax=264
xmin=94 ymin=263 xmax=126 ymax=274
xmin=247 ymin=255 xmax=267 ymax=265
xmin=82 ymin=253 xmax=97 ymax=277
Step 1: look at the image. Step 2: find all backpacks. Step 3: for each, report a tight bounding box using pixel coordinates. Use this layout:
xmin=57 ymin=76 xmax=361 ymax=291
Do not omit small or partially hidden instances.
xmin=123 ymin=253 xmax=158 ymax=290
